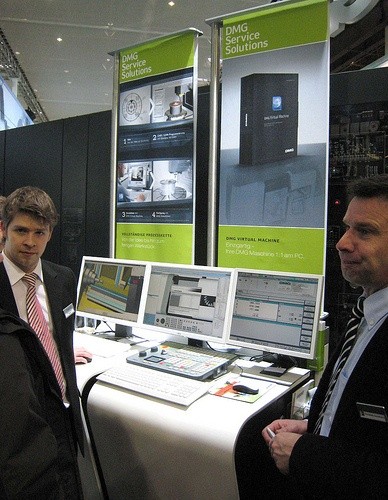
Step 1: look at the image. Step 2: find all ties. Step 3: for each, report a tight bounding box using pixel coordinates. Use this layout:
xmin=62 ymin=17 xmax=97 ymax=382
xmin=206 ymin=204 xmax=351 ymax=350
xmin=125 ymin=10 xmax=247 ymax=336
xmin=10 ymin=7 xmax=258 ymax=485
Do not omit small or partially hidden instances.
xmin=22 ymin=273 xmax=65 ymax=400
xmin=311 ymin=297 xmax=366 ymax=436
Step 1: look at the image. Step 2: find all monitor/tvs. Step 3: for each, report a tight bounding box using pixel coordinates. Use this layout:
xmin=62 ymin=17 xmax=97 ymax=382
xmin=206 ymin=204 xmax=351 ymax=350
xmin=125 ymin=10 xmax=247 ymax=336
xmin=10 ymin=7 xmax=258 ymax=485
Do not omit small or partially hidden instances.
xmin=127 ymin=163 xmax=148 ymax=192
xmin=75 ymin=255 xmax=324 ymax=377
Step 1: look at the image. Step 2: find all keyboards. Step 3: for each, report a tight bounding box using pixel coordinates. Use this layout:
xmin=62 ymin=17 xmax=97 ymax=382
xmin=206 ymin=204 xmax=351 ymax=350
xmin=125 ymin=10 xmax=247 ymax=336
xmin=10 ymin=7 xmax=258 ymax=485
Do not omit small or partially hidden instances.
xmin=95 ymin=363 xmax=212 ymax=407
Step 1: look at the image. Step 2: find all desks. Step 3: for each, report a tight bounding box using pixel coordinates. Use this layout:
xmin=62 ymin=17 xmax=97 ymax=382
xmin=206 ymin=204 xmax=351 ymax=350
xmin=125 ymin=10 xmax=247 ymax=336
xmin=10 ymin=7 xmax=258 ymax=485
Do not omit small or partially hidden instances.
xmin=73 ymin=319 xmax=311 ymax=500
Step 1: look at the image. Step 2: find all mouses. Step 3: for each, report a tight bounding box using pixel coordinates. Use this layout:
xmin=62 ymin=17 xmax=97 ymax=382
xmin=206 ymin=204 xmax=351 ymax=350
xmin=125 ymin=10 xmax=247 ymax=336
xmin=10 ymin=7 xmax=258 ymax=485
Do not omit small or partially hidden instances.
xmin=233 ymin=382 xmax=259 ymax=394
xmin=79 ymin=357 xmax=92 ymax=364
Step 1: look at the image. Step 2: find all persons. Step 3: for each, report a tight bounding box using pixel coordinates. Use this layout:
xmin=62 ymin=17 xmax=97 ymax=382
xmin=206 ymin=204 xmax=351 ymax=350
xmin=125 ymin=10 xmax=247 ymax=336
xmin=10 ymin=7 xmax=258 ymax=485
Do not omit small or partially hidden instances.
xmin=0 ymin=186 xmax=93 ymax=500
xmin=262 ymin=177 xmax=388 ymax=500
